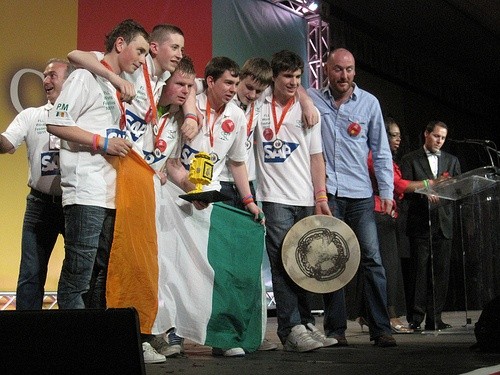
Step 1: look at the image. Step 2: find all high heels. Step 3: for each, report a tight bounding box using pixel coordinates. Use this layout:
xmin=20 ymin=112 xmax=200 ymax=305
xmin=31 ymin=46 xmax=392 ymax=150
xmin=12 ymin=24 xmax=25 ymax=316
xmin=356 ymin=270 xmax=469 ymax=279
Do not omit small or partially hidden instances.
xmin=389 ymin=321 xmax=414 ymax=333
xmin=358 ymin=317 xmax=370 ymax=331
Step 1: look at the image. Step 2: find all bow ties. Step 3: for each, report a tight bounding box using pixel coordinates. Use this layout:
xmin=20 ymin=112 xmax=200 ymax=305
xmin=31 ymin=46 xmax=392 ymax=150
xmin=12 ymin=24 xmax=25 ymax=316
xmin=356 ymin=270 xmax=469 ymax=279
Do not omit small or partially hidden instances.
xmin=426 ymin=151 xmax=441 ymax=157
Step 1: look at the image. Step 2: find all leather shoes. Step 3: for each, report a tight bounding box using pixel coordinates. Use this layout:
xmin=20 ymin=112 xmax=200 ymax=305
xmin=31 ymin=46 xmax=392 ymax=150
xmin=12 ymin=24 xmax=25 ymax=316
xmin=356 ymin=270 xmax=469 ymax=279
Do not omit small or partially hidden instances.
xmin=327 ymin=331 xmax=348 ymax=346
xmin=374 ymin=335 xmax=396 ymax=346
xmin=424 ymin=322 xmax=451 ymax=330
xmin=409 ymin=322 xmax=421 ymax=329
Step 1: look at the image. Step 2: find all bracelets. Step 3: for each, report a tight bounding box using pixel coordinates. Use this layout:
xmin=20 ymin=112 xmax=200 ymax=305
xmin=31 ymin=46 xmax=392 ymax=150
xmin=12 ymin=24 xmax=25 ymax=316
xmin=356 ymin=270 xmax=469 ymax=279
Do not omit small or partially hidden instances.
xmin=102 ymin=137 xmax=109 ymax=151
xmin=184 ymin=112 xmax=198 ymax=121
xmin=240 ymin=193 xmax=254 ymax=205
xmin=422 ymin=178 xmax=430 ymax=188
xmin=313 ymin=189 xmax=329 ymax=202
xmin=92 ymin=134 xmax=102 ymax=150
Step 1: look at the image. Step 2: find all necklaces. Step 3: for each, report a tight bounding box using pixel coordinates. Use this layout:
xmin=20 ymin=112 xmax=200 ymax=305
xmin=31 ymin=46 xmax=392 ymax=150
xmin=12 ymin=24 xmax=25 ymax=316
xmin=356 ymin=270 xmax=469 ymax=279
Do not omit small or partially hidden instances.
xmin=366 ymin=120 xmax=440 ymax=334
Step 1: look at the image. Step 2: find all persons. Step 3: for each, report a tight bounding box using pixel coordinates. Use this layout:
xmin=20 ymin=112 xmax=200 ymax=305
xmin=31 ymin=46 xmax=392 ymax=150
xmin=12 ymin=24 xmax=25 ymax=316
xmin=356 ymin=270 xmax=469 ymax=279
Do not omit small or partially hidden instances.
xmin=303 ymin=48 xmax=398 ymax=347
xmin=1 ymin=59 xmax=78 ymax=311
xmin=46 ymin=17 xmax=342 ymax=359
xmin=400 ymin=122 xmax=462 ymax=330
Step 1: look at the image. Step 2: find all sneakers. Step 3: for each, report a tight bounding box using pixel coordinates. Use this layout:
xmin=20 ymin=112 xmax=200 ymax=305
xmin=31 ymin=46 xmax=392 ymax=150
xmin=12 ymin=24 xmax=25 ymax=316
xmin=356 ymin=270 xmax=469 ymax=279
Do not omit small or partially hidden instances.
xmin=150 ymin=336 xmax=175 ymax=357
xmin=285 ymin=325 xmax=324 ymax=352
xmin=306 ymin=323 xmax=338 ymax=347
xmin=218 ymin=347 xmax=245 ymax=356
xmin=256 ymin=337 xmax=278 ymax=351
xmin=142 ymin=342 xmax=166 ymax=363
xmin=166 ymin=331 xmax=184 ymax=353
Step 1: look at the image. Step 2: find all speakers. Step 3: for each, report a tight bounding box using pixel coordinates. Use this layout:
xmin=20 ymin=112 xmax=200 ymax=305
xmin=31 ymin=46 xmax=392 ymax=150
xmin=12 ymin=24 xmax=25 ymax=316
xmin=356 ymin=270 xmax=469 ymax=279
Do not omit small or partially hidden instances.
xmin=0 ymin=307 xmax=146 ymax=375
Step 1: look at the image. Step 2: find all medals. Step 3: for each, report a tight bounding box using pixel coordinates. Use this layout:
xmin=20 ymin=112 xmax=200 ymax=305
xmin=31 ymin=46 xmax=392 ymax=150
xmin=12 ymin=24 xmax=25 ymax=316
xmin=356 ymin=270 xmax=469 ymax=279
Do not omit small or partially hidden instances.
xmin=246 ymin=138 xmax=252 ymax=151
xmin=152 ymin=147 xmax=163 ymax=159
xmin=150 ymin=123 xmax=160 ymax=137
xmin=207 ymin=150 xmax=219 ymax=165
xmin=272 ymin=138 xmax=283 ymax=150
